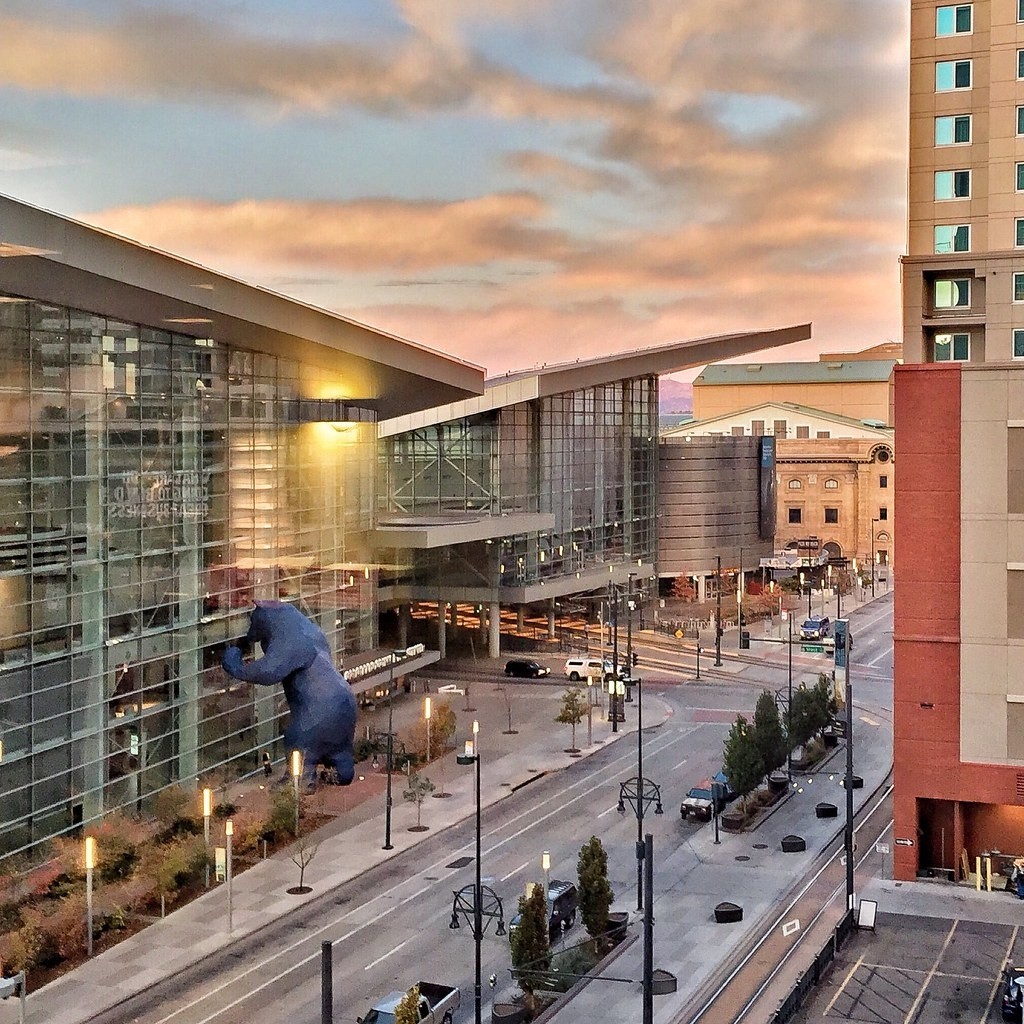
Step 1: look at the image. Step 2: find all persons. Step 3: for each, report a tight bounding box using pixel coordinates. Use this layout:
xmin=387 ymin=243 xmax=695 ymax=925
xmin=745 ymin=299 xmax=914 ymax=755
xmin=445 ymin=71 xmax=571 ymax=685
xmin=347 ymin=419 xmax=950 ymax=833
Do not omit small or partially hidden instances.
xmin=263 ymin=748 xmax=272 ymax=777
xmin=584 ymin=622 xmax=590 ymax=640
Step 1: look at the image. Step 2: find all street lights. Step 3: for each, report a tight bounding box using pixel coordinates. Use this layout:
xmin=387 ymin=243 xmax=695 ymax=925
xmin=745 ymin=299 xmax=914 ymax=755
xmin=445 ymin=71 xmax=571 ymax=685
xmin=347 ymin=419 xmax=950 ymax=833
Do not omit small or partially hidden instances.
xmin=449 ymin=752 xmax=506 ymax=1024
xmin=713 ymin=554 xmax=723 ymax=666
xmin=627 ymin=572 xmax=637 ymax=678
xmin=740 ymin=546 xmax=751 ymax=648
xmin=616 ymin=677 xmax=665 ymax=914
xmin=872 ymin=519 xmax=879 ymax=596
xmin=383 ymin=650 xmax=394 ymax=849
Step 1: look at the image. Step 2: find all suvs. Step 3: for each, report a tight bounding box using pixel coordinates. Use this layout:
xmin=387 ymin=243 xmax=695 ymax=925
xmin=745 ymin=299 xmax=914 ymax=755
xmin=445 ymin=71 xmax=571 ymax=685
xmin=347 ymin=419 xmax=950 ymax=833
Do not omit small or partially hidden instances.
xmin=509 ymin=880 xmax=579 ymax=943
xmin=503 ymin=659 xmax=551 ymax=678
xmin=800 ymin=616 xmax=830 ymax=640
xmin=680 ymin=781 xmax=727 ymax=822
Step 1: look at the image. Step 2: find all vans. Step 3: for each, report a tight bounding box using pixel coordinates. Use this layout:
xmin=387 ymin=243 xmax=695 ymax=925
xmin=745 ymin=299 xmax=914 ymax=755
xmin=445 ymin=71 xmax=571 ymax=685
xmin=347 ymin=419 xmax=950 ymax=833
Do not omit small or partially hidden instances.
xmin=564 ymin=659 xmax=626 ymax=681
